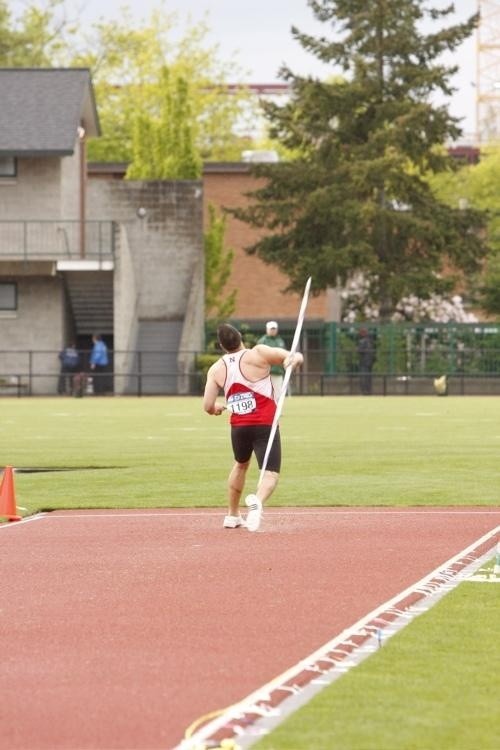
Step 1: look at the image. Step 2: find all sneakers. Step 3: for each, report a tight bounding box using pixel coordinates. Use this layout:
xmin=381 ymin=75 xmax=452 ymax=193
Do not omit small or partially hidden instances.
xmin=223 ymin=515 xmax=243 ymax=529
xmin=245 ymin=494 xmax=262 ymax=531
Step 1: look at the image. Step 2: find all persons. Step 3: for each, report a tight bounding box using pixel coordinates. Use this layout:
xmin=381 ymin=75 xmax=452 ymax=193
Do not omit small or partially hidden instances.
xmin=58 ymin=340 xmax=84 ymax=398
xmin=357 ymin=328 xmax=376 ymax=394
xmin=90 ymin=334 xmax=110 ymax=394
xmin=256 ymin=320 xmax=288 ymax=417
xmin=202 ymin=322 xmax=304 ymax=533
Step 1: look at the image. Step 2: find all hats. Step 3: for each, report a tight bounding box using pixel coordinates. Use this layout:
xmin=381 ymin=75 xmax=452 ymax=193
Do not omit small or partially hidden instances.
xmin=266 ymin=321 xmax=277 ymax=328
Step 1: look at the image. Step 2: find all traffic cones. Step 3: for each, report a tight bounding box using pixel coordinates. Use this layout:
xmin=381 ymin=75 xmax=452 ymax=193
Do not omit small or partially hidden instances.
xmin=0 ymin=464 xmax=22 ymax=521
